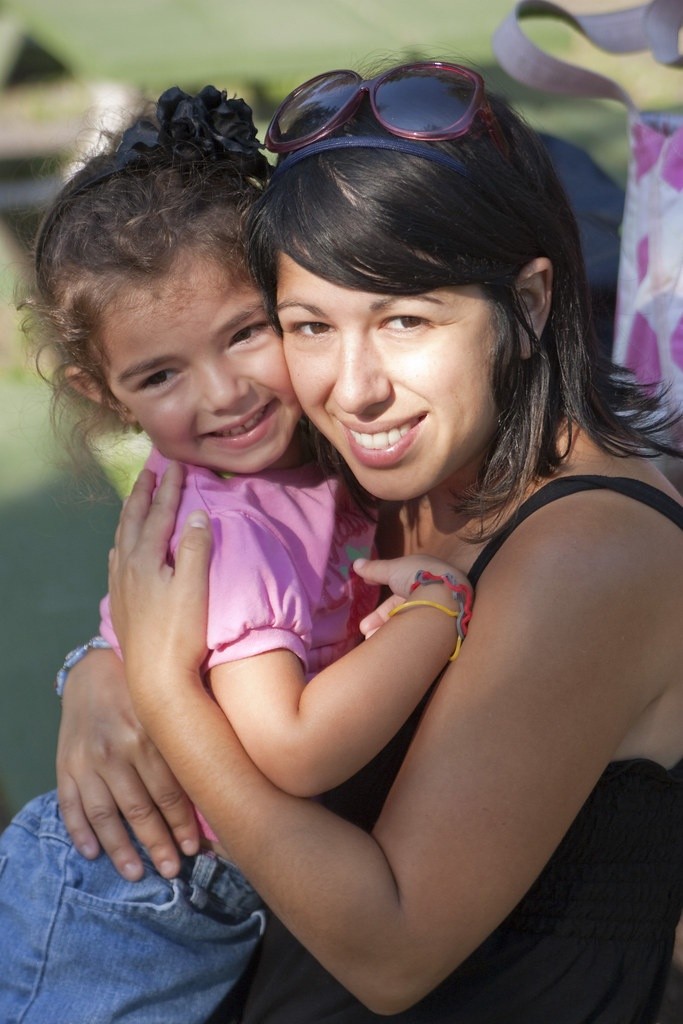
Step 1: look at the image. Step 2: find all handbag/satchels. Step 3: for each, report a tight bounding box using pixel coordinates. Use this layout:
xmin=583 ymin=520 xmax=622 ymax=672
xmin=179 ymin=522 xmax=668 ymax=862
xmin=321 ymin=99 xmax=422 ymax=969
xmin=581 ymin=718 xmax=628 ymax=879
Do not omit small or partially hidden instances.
xmin=605 ymin=114 xmax=683 ymax=429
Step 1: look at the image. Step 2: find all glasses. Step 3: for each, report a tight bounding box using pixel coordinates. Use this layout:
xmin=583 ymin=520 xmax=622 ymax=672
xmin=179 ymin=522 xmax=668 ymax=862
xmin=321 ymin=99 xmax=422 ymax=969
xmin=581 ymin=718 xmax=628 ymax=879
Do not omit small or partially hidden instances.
xmin=265 ymin=62 xmax=512 ymax=166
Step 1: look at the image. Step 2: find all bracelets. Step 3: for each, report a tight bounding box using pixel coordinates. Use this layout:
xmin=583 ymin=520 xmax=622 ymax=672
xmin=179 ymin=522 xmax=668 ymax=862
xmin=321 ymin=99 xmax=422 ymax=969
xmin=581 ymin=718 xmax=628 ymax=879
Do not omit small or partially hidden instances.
xmin=388 ymin=569 xmax=473 ymax=662
xmin=53 ymin=635 xmax=114 ymax=705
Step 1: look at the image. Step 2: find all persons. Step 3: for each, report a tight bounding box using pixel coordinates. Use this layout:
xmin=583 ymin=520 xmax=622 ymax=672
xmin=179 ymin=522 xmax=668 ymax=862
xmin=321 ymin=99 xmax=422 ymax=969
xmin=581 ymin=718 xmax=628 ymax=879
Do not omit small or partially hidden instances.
xmin=0 ymin=55 xmax=683 ymax=1024
xmin=0 ymin=85 xmax=475 ymax=1024
